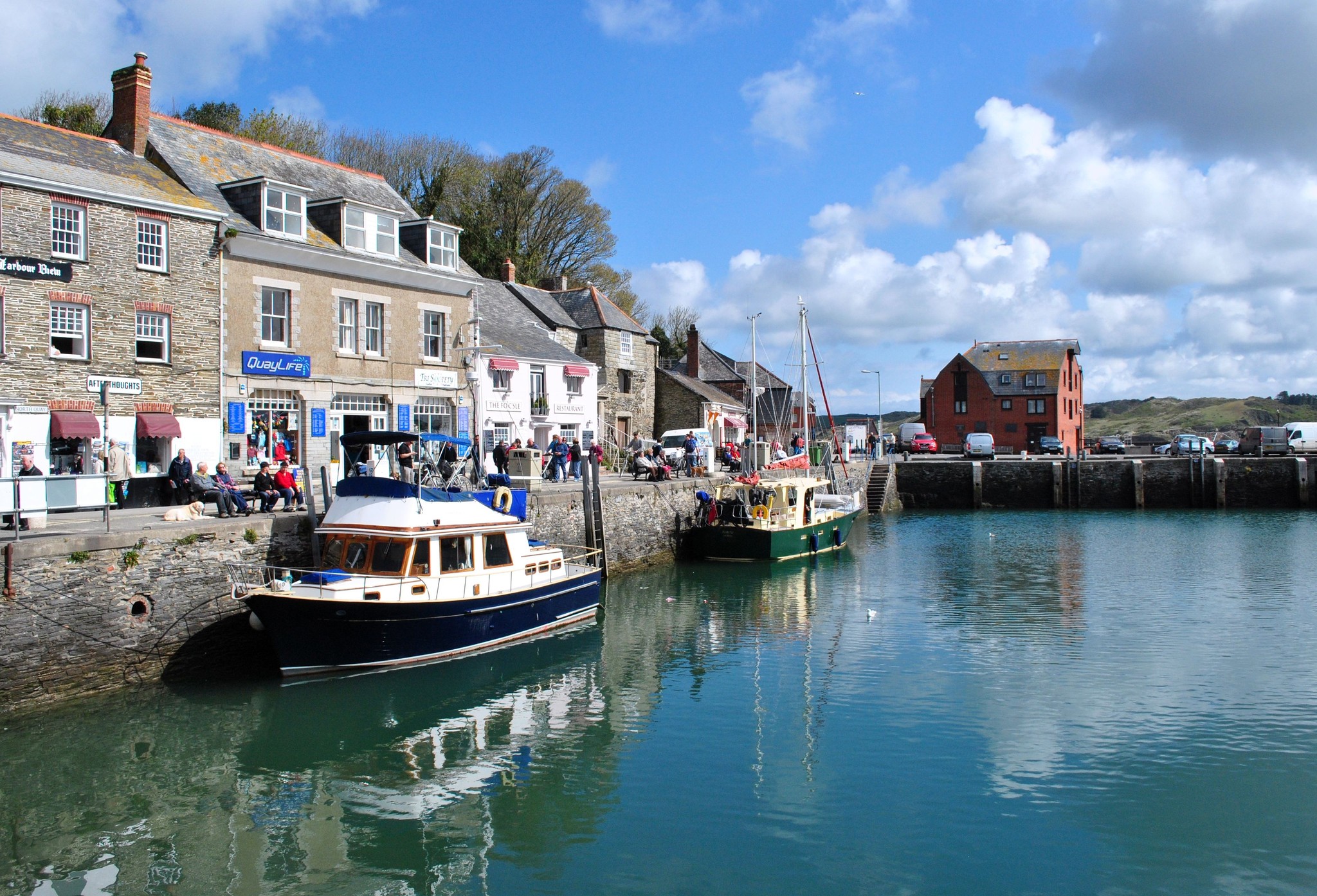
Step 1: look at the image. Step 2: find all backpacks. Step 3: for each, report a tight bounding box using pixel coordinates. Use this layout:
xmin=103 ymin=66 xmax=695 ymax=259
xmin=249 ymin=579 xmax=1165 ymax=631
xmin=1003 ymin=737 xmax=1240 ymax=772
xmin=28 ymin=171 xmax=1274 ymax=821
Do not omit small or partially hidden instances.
xmin=685 ymin=440 xmax=695 ymax=453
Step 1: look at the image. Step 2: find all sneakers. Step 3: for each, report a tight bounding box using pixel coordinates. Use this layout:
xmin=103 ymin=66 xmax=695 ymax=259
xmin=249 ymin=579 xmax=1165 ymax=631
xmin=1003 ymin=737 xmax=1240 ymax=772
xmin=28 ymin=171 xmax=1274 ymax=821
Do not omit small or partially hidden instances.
xmin=0 ymin=524 xmax=15 ymax=530
xmin=219 ymin=512 xmax=230 ymax=518
xmin=18 ymin=524 xmax=29 ymax=530
xmin=230 ymin=511 xmax=240 ymax=517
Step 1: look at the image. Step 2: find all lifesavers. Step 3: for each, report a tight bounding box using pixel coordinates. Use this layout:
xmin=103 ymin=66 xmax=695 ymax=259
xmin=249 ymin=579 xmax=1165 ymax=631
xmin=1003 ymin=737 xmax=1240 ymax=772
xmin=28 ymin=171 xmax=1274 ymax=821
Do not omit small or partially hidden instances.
xmin=492 ymin=486 xmax=513 ymax=514
xmin=752 ymin=505 xmax=769 ymax=520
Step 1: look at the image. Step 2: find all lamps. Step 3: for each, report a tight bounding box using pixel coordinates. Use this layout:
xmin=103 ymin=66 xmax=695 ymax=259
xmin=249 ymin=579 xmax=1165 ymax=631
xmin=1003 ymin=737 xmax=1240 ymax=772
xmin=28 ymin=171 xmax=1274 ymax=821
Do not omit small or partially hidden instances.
xmin=521 ymin=418 xmax=527 ymax=423
xmin=569 ymin=395 xmax=575 ymax=399
xmin=587 ymin=420 xmax=593 ymax=424
xmin=459 ymin=317 xmax=482 ymax=342
xmin=487 ymin=417 xmax=493 ymax=421
xmin=503 ymin=392 xmax=510 ymax=396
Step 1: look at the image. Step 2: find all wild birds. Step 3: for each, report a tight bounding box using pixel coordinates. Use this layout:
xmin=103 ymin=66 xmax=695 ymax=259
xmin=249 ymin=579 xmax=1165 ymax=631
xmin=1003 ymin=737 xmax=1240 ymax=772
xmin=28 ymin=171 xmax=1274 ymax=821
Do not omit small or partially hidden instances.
xmin=865 ymin=609 xmax=877 ymax=617
xmin=989 ymin=533 xmax=996 ymax=538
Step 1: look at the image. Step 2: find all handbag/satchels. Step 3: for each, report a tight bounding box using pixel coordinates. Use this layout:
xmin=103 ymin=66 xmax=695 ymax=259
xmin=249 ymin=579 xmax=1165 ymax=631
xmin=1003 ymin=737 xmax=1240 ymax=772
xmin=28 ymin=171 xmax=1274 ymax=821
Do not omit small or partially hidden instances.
xmin=597 ymin=455 xmax=603 ymax=463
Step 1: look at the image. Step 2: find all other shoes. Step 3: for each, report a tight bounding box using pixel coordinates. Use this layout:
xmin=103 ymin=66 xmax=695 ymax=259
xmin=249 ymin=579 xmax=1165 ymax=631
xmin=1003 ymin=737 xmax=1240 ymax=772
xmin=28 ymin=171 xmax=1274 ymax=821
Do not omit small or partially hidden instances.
xmin=574 ymin=480 xmax=579 ymax=482
xmin=245 ymin=507 xmax=256 ymax=516
xmin=261 ymin=509 xmax=274 ymax=513
xmin=283 ymin=507 xmax=296 ymax=512
xmin=730 ymin=470 xmax=742 ymax=472
xmin=563 ymin=479 xmax=567 ymax=482
xmin=552 ymin=480 xmax=558 ymax=483
xmin=111 ymin=506 xmax=124 ymax=510
xmin=653 ymin=476 xmax=675 ymax=482
xmin=688 ymin=475 xmax=697 ymax=477
xmin=298 ymin=506 xmax=307 ymax=511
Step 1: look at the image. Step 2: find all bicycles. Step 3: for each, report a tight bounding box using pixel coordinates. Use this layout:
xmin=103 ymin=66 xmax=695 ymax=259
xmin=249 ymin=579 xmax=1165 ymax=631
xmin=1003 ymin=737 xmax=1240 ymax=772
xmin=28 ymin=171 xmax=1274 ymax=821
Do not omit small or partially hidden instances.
xmin=673 ymin=448 xmax=704 ymax=476
xmin=387 ymin=461 xmax=448 ymax=491
xmin=421 ymin=459 xmax=473 ymax=493
xmin=620 ymin=448 xmax=641 ymax=478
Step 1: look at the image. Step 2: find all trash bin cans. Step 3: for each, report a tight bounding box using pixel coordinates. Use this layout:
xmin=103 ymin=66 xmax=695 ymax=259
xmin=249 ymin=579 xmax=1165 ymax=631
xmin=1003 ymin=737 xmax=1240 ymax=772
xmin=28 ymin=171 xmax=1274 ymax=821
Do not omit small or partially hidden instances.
xmin=814 ymin=441 xmax=832 ymax=465
xmin=508 ymin=448 xmax=543 ymax=493
xmin=809 ymin=447 xmax=824 ymax=466
xmin=750 ymin=441 xmax=770 ymax=469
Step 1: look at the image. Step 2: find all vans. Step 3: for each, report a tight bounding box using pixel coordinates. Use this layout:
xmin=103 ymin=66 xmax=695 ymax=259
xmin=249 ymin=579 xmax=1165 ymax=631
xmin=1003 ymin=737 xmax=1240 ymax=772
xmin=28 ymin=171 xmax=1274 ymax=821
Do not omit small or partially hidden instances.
xmin=896 ymin=422 xmax=926 ymax=453
xmin=660 ymin=428 xmax=713 ymax=470
xmin=1282 ymin=422 xmax=1317 ymax=454
xmin=1238 ymin=426 xmax=1289 ymax=455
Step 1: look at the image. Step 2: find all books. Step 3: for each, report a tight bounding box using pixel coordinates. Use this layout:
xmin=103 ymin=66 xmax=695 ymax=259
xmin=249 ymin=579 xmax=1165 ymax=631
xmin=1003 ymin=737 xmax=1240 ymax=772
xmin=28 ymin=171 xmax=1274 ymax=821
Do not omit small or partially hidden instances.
xmin=170 ymin=481 xmax=177 ymax=489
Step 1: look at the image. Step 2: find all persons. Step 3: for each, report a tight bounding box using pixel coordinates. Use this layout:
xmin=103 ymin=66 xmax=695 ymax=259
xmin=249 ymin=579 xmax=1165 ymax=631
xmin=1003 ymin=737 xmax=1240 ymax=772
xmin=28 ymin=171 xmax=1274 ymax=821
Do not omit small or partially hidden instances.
xmin=108 ymin=438 xmax=129 ymax=510
xmin=436 ymin=427 xmax=896 ymax=491
xmin=804 ymin=491 xmax=813 ymax=523
xmin=254 ymin=461 xmax=280 ymax=513
xmin=168 ymin=449 xmax=193 ymax=506
xmin=1 ymin=454 xmax=43 ymax=531
xmin=94 ymin=450 xmax=104 ymax=475
xmin=398 ymin=441 xmax=417 ymax=485
xmin=274 ymin=462 xmax=307 ymax=512
xmin=190 ymin=462 xmax=238 ymax=519
xmin=212 ymin=462 xmax=256 ymax=517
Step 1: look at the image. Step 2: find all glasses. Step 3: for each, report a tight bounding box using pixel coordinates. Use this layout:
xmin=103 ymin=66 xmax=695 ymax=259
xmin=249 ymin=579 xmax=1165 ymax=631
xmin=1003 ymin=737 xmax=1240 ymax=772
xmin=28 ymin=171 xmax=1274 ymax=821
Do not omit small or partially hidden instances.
xmin=553 ymin=437 xmax=556 ymax=438
xmin=409 ymin=441 xmax=413 ymax=444
xmin=563 ymin=440 xmax=566 ymax=441
xmin=634 ymin=434 xmax=637 ymax=435
xmin=219 ymin=466 xmax=225 ymax=468
xmin=685 ymin=436 xmax=689 ymax=437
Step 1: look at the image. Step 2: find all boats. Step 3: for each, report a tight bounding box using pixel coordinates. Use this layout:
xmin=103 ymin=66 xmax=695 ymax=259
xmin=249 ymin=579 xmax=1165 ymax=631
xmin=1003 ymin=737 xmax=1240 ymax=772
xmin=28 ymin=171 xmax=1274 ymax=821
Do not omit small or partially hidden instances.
xmin=674 ymin=296 xmax=864 ymax=564
xmin=225 ymin=430 xmax=603 ymax=677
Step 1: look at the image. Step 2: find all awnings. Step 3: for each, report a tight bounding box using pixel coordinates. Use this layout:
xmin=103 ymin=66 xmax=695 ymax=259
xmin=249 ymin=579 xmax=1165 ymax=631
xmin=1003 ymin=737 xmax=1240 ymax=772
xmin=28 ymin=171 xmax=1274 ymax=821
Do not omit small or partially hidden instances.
xmin=50 ymin=411 xmax=101 ymax=440
xmin=489 ymin=358 xmax=519 ymax=371
xmin=564 ymin=365 xmax=590 ymax=377
xmin=137 ymin=413 xmax=181 ymax=440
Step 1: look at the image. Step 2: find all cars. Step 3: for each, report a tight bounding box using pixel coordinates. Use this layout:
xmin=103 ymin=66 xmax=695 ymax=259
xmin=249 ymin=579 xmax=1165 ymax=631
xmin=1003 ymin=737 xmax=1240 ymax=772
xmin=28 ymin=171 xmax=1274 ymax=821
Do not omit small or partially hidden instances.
xmin=961 ymin=433 xmax=995 ymax=460
xmin=1155 ymin=437 xmax=1214 ymax=454
xmin=1096 ymin=437 xmax=1126 ymax=454
xmin=1170 ymin=434 xmax=1206 ymax=456
xmin=911 ymin=433 xmax=937 ymax=455
xmin=1214 ymin=440 xmax=1239 ymax=454
xmin=1033 ymin=436 xmax=1064 ymax=454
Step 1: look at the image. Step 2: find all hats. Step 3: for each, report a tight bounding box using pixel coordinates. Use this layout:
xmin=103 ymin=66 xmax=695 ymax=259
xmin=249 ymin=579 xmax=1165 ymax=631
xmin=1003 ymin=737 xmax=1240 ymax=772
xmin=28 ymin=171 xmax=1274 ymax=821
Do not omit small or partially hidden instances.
xmin=633 ymin=431 xmax=638 ymax=434
xmin=260 ymin=462 xmax=272 ymax=466
xmin=280 ymin=462 xmax=289 ymax=466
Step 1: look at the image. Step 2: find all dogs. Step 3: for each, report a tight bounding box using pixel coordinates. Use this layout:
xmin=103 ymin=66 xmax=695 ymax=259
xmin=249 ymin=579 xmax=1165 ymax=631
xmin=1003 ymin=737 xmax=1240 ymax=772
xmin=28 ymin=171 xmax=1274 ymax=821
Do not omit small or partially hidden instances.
xmin=163 ymin=501 xmax=216 ymax=521
xmin=689 ymin=463 xmax=709 ymax=479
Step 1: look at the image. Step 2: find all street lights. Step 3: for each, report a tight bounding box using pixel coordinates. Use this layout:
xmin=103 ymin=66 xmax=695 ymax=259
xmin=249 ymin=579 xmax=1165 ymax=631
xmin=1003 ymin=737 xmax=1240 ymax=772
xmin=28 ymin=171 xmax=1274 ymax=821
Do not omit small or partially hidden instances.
xmin=862 ymin=370 xmax=884 ymax=458
xmin=458 ymin=316 xmax=483 ymax=344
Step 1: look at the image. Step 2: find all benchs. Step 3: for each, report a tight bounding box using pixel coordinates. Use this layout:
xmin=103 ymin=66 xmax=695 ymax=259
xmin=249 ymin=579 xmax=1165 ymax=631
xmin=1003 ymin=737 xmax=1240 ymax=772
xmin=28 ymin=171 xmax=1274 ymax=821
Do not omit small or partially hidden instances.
xmin=186 ymin=484 xmax=297 ymax=516
xmin=720 ymin=456 xmax=731 ymax=472
xmin=633 ymin=458 xmax=680 ymax=482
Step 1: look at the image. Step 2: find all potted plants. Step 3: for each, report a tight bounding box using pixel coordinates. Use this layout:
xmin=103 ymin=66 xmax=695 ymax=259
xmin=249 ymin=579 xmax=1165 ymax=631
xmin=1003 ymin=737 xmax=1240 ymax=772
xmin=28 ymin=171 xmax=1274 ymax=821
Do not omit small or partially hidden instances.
xmin=531 ymin=397 xmax=549 ymax=415
